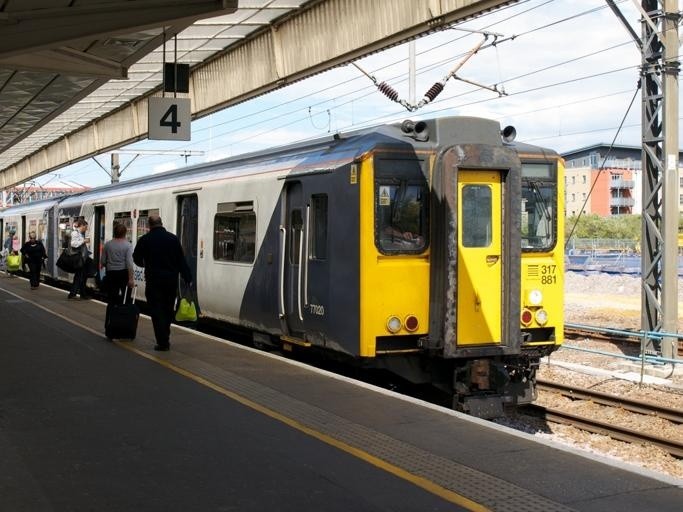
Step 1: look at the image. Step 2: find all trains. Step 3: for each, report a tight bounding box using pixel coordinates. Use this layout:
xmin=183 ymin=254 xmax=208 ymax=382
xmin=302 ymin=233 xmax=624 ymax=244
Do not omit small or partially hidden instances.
xmin=0 ymin=116 xmax=571 ymax=418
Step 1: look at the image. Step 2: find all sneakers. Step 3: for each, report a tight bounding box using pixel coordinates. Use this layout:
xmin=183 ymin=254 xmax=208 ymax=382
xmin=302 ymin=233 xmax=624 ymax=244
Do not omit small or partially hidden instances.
xmin=154 ymin=342 xmax=169 ymax=351
xmin=67 ymin=295 xmax=80 ymax=301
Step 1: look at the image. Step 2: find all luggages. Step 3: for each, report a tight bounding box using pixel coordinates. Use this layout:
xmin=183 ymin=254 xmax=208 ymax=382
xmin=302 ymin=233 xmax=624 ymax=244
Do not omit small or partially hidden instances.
xmin=104 ymin=285 xmax=140 ymax=341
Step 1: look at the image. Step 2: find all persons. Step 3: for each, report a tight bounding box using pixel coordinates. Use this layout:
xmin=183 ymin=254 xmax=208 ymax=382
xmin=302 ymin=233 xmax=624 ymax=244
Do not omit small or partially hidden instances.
xmin=100 ymin=224 xmax=135 ymax=305
xmin=67 ymin=219 xmax=95 ymax=300
xmin=382 ymin=223 xmax=413 ymax=241
xmin=0 ymin=222 xmax=48 ymax=290
xmin=131 ymin=214 xmax=194 ymax=351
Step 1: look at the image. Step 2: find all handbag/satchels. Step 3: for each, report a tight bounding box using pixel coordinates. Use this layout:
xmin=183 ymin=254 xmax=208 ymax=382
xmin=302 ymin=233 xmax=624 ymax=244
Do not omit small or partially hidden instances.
xmin=6 ymin=255 xmax=20 ymax=273
xmin=173 ymin=297 xmax=197 ymax=322
xmin=55 ymin=250 xmax=80 ymax=273
xmin=86 ymin=257 xmax=97 ymax=279
